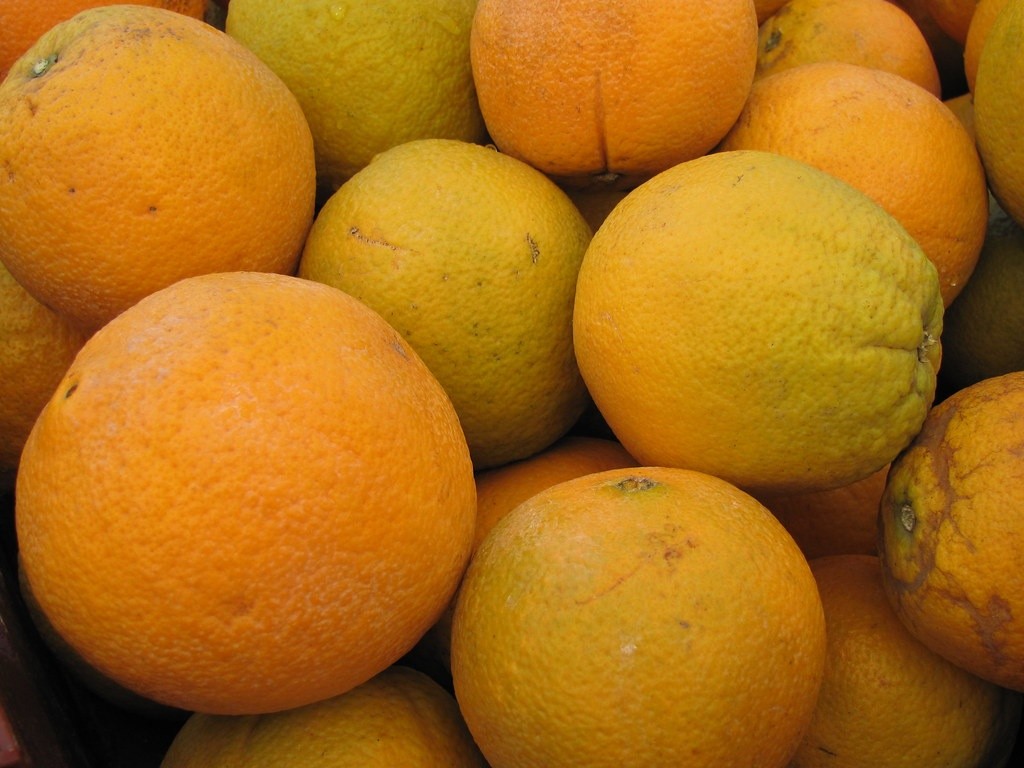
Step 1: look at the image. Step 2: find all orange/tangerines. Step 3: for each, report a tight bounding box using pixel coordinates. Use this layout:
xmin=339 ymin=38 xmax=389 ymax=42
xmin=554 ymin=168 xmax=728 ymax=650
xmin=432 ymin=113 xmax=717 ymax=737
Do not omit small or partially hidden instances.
xmin=0 ymin=0 xmax=1024 ymax=768
